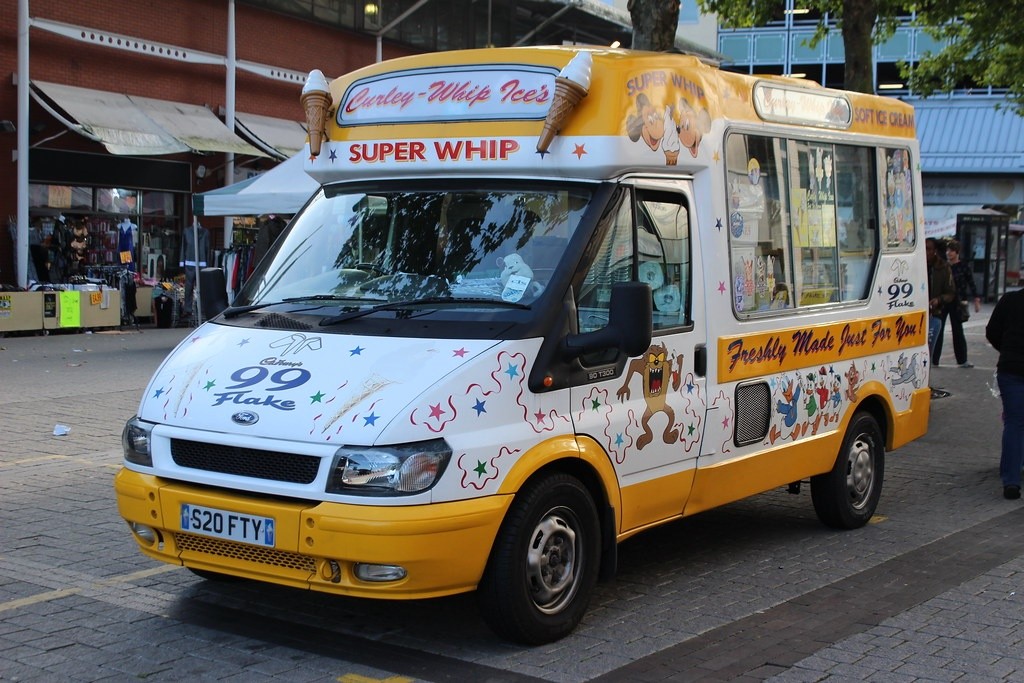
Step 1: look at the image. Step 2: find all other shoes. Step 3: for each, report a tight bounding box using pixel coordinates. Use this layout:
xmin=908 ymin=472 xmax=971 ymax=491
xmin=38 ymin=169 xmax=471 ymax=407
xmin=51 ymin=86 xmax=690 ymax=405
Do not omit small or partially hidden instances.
xmin=1004 ymin=484 xmax=1021 ymax=499
xmin=957 ymin=360 xmax=974 ymax=368
xmin=931 ymin=361 xmax=939 ymax=368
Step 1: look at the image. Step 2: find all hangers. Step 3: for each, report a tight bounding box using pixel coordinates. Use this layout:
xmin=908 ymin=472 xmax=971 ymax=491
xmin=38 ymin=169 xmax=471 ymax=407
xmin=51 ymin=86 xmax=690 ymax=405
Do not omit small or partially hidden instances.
xmin=226 ymin=243 xmax=255 ymax=254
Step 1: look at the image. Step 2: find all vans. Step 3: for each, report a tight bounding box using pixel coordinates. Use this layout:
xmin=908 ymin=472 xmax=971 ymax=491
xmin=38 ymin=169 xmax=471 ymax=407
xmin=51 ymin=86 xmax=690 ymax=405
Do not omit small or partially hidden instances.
xmin=115 ymin=45 xmax=931 ymax=648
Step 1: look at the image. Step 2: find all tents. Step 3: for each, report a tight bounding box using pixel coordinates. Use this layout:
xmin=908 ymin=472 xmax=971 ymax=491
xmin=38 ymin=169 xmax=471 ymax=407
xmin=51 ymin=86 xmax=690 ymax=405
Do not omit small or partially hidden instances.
xmin=192 ymin=148 xmax=321 ymax=327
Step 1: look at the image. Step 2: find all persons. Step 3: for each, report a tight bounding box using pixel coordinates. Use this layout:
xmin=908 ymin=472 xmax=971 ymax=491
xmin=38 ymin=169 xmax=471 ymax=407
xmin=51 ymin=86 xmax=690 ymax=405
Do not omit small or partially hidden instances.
xmin=117 ymin=218 xmax=137 ymax=268
xmin=932 ymin=238 xmax=980 ymax=369
xmin=985 ymin=288 xmax=1024 ymax=500
xmin=925 ymin=237 xmax=957 ymax=372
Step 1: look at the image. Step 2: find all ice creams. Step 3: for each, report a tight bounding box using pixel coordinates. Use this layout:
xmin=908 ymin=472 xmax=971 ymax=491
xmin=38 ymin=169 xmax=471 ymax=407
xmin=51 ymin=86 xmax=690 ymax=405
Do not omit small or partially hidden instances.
xmin=536 ymin=51 xmax=593 ymax=152
xmin=300 ymin=69 xmax=332 ymax=156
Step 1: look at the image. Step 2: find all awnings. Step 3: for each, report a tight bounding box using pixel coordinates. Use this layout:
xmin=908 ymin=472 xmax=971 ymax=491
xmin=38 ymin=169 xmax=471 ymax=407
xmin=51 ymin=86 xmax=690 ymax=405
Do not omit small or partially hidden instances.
xmin=28 ymin=80 xmax=277 ymax=180
xmin=922 ymin=205 xmax=983 ymax=239
xmin=234 ymin=111 xmax=308 ymax=160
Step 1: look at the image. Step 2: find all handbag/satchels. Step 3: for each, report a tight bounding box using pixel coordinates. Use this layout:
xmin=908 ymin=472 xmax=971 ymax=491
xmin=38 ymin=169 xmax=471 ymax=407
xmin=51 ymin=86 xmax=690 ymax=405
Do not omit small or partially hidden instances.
xmin=958 ymin=300 xmax=969 ymax=322
